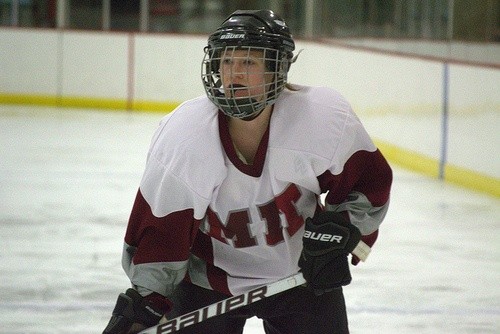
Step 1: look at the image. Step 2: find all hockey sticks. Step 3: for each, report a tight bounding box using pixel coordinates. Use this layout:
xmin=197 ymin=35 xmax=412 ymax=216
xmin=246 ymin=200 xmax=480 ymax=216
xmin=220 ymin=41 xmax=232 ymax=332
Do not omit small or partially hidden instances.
xmin=133 ymin=248 xmax=353 ymax=334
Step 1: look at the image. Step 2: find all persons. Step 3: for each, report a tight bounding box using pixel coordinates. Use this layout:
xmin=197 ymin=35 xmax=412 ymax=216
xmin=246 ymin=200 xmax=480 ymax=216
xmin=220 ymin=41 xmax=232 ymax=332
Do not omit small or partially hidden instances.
xmin=103 ymin=8 xmax=393 ymax=334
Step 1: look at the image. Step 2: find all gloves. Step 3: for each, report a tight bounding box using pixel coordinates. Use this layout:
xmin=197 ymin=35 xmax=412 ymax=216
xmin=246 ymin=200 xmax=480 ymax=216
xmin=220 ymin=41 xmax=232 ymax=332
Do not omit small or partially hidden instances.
xmin=102 ymin=288 xmax=170 ymax=334
xmin=298 ymin=210 xmax=361 ymax=297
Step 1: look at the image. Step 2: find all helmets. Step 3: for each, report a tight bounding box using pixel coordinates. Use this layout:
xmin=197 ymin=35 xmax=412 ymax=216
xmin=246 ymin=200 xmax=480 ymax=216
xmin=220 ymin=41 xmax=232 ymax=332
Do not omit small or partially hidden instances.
xmin=208 ymin=9 xmax=295 ymax=73
